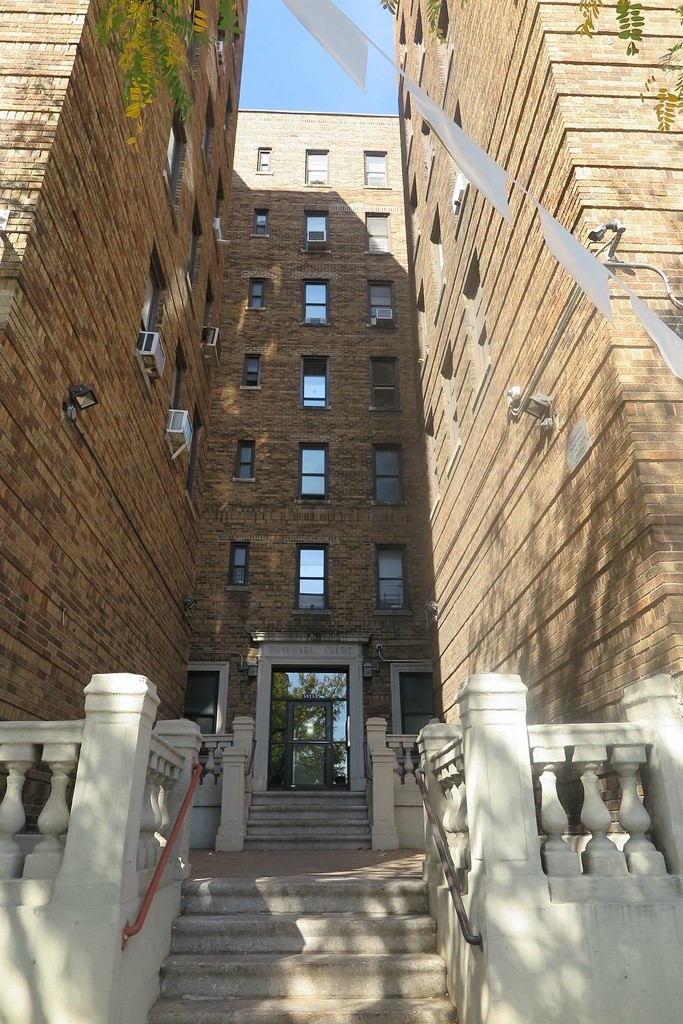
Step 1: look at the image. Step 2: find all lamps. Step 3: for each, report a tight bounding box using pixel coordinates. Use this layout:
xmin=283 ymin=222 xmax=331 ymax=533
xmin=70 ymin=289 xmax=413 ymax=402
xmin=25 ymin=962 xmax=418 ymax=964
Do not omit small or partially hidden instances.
xmin=247 ymin=660 xmax=259 ymax=677
xmin=62 ymin=384 xmax=99 ymax=419
xmin=524 ymin=396 xmax=553 ymax=428
xmin=424 ymin=601 xmax=437 ymax=614
xmin=181 ymin=598 xmax=196 ymax=616
xmin=362 ymin=660 xmax=378 ymax=678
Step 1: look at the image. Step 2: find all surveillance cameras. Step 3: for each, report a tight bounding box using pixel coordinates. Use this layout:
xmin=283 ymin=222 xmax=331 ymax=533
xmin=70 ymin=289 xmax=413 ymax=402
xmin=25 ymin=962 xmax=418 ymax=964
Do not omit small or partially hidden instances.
xmin=376 ymin=644 xmax=383 ymax=650
xmin=587 ymin=225 xmax=608 ymax=242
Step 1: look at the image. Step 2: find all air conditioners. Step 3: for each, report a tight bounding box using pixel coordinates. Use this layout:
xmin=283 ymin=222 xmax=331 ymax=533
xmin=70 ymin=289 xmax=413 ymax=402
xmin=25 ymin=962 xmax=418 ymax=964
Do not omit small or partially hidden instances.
xmin=375 ymin=307 xmax=394 ymax=319
xmin=214 ymin=217 xmax=223 ymax=241
xmin=216 ymin=40 xmax=226 ymax=76
xmin=307 ymin=229 xmax=327 ymax=244
xmin=166 ymin=407 xmax=194 ymax=453
xmin=135 ymin=330 xmax=166 ymax=380
xmin=202 ymin=326 xmax=222 ymax=366
xmin=452 ymin=175 xmax=465 ymax=216
